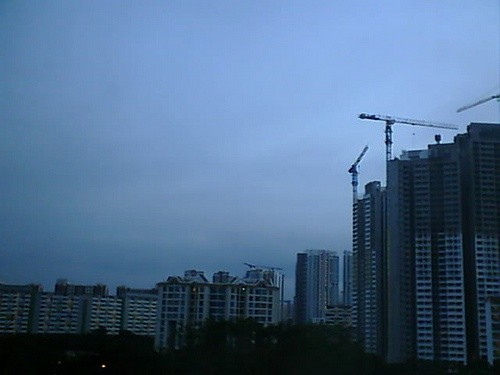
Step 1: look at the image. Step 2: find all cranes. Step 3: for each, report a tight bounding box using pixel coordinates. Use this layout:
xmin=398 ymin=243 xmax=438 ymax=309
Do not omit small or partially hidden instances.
xmin=357 ymin=113 xmax=459 ymax=187
xmin=457 ymin=94 xmax=500 ymax=113
xmin=348 ymin=145 xmax=369 ymax=201
